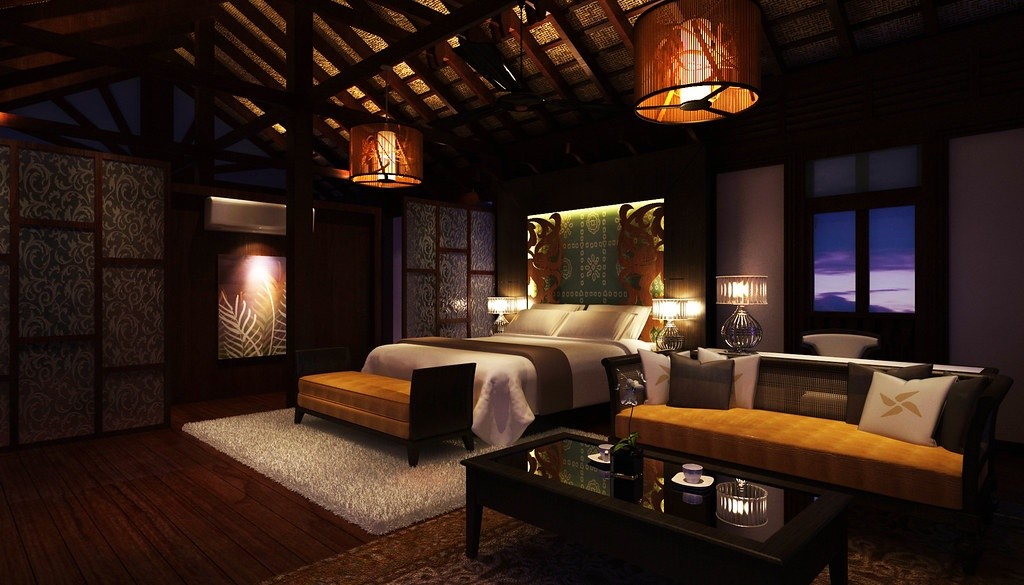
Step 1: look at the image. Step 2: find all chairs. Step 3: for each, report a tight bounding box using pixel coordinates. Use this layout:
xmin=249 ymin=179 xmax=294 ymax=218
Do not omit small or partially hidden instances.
xmin=802 ymin=335 xmax=879 ymax=419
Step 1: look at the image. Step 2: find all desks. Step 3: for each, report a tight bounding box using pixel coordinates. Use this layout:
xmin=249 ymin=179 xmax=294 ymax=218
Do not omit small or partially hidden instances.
xmin=690 ymin=346 xmax=1000 ymax=377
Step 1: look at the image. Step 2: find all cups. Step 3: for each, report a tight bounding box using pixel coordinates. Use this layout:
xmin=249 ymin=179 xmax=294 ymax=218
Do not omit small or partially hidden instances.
xmin=682 ymin=464 xmax=703 ymax=484
xmin=599 ymin=444 xmax=614 ymax=461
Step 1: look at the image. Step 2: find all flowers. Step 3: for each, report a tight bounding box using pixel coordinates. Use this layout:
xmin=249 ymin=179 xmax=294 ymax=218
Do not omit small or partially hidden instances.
xmin=612 ymin=368 xmax=647 ymax=454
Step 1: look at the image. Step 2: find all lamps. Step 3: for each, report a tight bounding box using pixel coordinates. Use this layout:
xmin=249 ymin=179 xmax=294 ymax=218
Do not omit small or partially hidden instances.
xmin=487 ymin=296 xmax=518 ymax=335
xmin=632 ymin=0 xmax=762 ymax=125
xmin=349 ymin=65 xmax=424 ymax=188
xmin=715 ymin=275 xmax=768 ymax=355
xmin=651 ymin=298 xmax=689 ymax=351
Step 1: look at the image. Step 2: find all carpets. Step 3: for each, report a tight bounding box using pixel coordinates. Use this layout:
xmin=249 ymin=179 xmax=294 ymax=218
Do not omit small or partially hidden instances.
xmin=249 ymin=498 xmax=1024 ymax=585
xmin=180 ymin=408 xmax=491 ymax=535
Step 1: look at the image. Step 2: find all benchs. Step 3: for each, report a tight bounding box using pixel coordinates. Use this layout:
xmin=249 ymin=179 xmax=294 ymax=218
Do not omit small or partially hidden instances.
xmin=600 ymin=350 xmax=1013 ymax=576
xmin=294 ymin=347 xmax=475 ymax=468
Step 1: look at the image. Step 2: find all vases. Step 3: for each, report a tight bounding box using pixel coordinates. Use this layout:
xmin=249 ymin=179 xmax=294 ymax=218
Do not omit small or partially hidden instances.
xmin=610 ymin=474 xmax=643 ymax=505
xmin=610 ymin=445 xmax=643 ymax=479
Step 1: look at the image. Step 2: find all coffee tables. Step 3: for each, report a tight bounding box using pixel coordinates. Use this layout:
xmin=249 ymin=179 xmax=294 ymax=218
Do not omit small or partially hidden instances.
xmin=462 ymin=433 xmax=852 ymax=585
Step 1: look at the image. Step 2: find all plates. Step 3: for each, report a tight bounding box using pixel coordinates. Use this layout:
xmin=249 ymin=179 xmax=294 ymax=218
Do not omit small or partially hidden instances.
xmin=670 ymin=472 xmax=714 ymax=488
xmin=587 ymin=454 xmax=610 ymax=464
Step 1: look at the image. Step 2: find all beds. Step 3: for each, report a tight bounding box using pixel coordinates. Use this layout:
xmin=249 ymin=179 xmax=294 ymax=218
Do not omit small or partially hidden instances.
xmin=362 ymin=330 xmax=629 ymax=440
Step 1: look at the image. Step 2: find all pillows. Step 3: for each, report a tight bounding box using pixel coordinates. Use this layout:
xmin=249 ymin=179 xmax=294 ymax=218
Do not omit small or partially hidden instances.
xmin=503 ymin=304 xmax=652 ymax=342
xmin=636 ymin=345 xmax=761 ymax=410
xmin=847 ymin=361 xmax=985 ymax=455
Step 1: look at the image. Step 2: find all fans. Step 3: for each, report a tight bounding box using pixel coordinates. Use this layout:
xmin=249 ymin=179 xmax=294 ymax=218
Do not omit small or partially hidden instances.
xmin=426 ymin=0 xmax=635 ymax=131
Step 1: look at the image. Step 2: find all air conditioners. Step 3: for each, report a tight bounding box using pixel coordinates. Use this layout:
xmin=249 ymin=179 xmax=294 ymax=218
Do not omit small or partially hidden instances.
xmin=205 ymin=196 xmax=316 ymax=235
